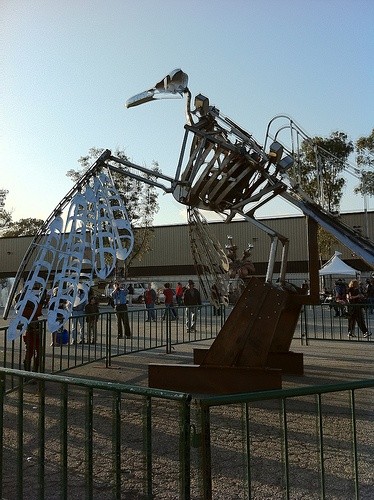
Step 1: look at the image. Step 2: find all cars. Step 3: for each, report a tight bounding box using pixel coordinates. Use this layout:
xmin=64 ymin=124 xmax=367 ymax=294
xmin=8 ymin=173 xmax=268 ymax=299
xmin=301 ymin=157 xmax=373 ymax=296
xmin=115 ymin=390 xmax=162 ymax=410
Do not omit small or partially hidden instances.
xmin=0 ymin=270 xmax=177 ymax=309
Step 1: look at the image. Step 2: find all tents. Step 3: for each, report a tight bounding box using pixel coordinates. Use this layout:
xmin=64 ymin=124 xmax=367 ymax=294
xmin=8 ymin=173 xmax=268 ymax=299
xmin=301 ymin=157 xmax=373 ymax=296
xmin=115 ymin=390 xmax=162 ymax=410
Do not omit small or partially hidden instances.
xmin=318 ymin=256 xmax=361 ymax=299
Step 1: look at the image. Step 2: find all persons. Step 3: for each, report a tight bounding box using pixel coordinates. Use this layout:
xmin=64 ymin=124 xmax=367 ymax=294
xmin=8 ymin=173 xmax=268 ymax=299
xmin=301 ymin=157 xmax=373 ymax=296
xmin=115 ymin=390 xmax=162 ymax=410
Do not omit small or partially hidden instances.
xmin=110 ymin=283 xmax=131 ymax=339
xmin=348 ymin=280 xmax=372 ymax=337
xmin=161 ymin=282 xmax=178 ymax=320
xmin=49 ymin=287 xmax=67 ymax=347
xmin=15 ymin=282 xmax=47 ymax=381
xmin=68 ymin=284 xmax=85 ymax=344
xmin=39 ymin=288 xmax=48 ymax=308
xmin=324 ymin=272 xmax=374 ymax=319
xmin=228 ymin=283 xmax=233 ymax=292
xmin=301 ymin=280 xmax=309 ymax=295
xmin=85 ymin=296 xmax=99 ymax=344
xmin=176 ymin=283 xmax=183 ymax=307
xmin=144 ymin=283 xmax=158 ymax=322
xmin=127 ymin=284 xmax=134 ymax=306
xmin=183 ymin=279 xmax=203 ymax=333
xmin=212 ymin=277 xmax=222 ymax=316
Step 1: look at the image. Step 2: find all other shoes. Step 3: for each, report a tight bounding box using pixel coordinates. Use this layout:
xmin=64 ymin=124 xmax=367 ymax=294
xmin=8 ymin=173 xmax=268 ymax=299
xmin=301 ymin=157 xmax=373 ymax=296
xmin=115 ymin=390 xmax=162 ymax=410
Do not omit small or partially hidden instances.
xmin=348 ymin=332 xmax=356 ymax=338
xmin=364 ymin=332 xmax=372 ymax=337
xmin=126 ymin=335 xmax=130 ymax=338
xmin=117 ymin=335 xmax=123 ymax=339
xmin=334 ymin=313 xmax=339 ymax=317
xmin=80 ymin=341 xmax=84 ymax=343
xmin=91 ymin=339 xmax=95 ymax=343
